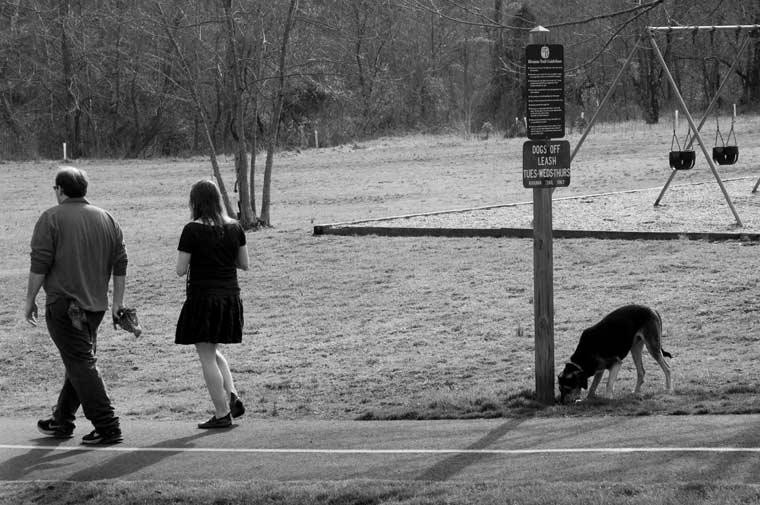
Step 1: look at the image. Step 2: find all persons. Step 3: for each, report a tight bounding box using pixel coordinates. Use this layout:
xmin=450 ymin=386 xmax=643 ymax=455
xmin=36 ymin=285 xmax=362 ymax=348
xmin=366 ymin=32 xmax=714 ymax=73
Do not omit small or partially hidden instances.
xmin=172 ymin=178 xmax=251 ymax=431
xmin=24 ymin=166 xmax=131 ymax=447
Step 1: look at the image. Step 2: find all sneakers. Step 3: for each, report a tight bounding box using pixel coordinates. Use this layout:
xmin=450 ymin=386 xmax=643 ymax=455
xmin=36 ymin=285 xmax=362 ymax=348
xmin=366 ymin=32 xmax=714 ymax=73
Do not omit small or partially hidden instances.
xmin=82 ymin=428 xmax=122 ymax=443
xmin=198 ymin=392 xmax=246 ymax=428
xmin=37 ymin=418 xmax=76 ymax=437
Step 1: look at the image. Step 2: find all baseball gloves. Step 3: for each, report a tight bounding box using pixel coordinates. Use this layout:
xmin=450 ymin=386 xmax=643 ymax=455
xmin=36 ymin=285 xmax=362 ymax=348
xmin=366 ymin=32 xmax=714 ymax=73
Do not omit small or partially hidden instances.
xmin=113 ymin=307 xmax=142 ymax=338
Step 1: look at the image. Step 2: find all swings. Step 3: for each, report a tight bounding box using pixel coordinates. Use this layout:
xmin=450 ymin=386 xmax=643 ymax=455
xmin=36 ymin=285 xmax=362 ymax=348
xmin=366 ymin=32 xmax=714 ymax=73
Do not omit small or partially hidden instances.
xmin=709 ymin=28 xmax=740 ymax=165
xmin=669 ymin=28 xmax=695 ymax=170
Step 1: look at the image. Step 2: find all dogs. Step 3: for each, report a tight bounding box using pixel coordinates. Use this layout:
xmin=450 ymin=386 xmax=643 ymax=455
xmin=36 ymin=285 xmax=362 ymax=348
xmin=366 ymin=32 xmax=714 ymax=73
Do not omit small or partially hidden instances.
xmin=558 ymin=303 xmax=674 ymax=406
